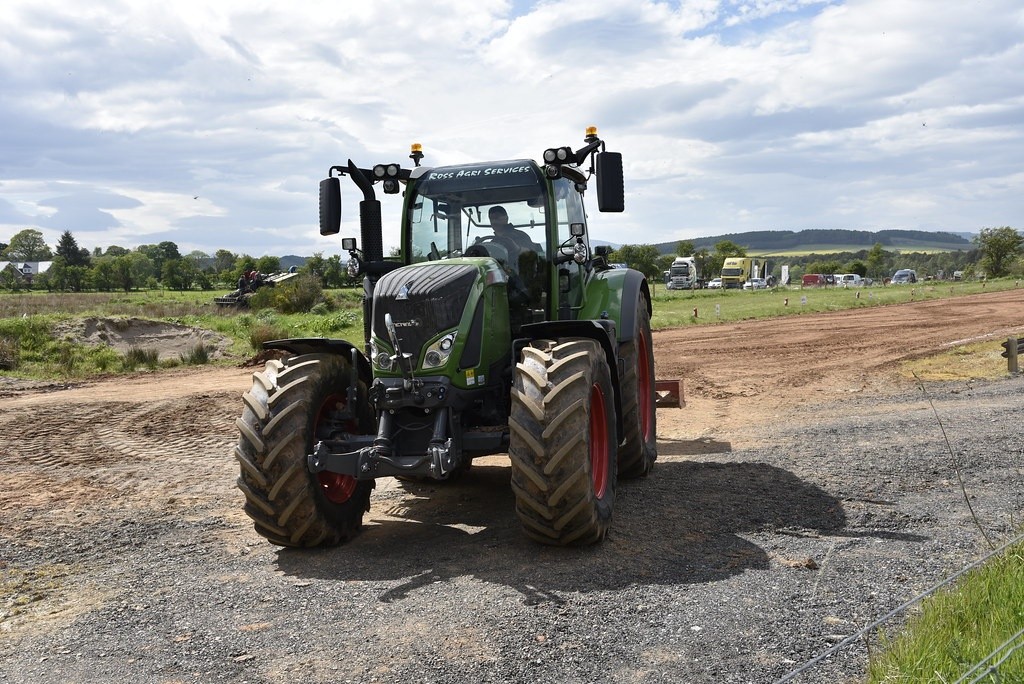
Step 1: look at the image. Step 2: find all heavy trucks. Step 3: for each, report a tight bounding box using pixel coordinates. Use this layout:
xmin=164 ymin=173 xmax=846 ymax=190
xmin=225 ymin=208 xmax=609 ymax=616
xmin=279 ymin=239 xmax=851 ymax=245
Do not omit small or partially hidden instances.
xmin=671 ymin=257 xmax=705 ymax=289
xmin=721 ymin=257 xmax=768 ymax=289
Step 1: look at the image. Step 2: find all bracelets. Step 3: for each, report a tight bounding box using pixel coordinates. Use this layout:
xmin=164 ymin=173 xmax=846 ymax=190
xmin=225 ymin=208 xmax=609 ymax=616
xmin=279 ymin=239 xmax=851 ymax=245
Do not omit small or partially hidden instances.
xmin=517 ymin=247 xmax=521 ymax=252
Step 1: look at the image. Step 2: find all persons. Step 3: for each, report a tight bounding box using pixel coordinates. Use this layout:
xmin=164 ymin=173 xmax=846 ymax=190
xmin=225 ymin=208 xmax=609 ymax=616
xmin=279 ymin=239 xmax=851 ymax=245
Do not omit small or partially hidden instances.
xmin=255 ymin=268 xmax=261 ymax=281
xmin=249 ymin=268 xmax=256 ymax=280
xmin=489 ymin=205 xmax=536 ymax=276
xmin=288 ymin=265 xmax=298 ymax=273
xmin=238 ymin=275 xmax=245 ymax=287
xmin=243 ymin=269 xmax=249 ymax=282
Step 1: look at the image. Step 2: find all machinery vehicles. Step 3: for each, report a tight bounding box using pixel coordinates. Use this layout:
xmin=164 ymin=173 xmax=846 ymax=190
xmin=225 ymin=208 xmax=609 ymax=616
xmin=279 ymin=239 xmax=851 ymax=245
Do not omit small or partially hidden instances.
xmin=234 ymin=125 xmax=686 ymax=549
xmin=214 ymin=266 xmax=300 ymax=306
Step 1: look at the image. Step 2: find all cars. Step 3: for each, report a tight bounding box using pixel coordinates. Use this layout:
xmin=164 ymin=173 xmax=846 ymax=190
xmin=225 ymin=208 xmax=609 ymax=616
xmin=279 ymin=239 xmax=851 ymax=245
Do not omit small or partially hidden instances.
xmin=743 ymin=275 xmax=777 ymax=289
xmin=704 ymin=278 xmax=722 ymax=289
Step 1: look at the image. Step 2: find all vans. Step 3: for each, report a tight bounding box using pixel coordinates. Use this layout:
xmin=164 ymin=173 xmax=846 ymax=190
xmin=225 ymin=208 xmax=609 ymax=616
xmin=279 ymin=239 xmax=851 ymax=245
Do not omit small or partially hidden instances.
xmin=800 ymin=269 xmax=947 ymax=287
